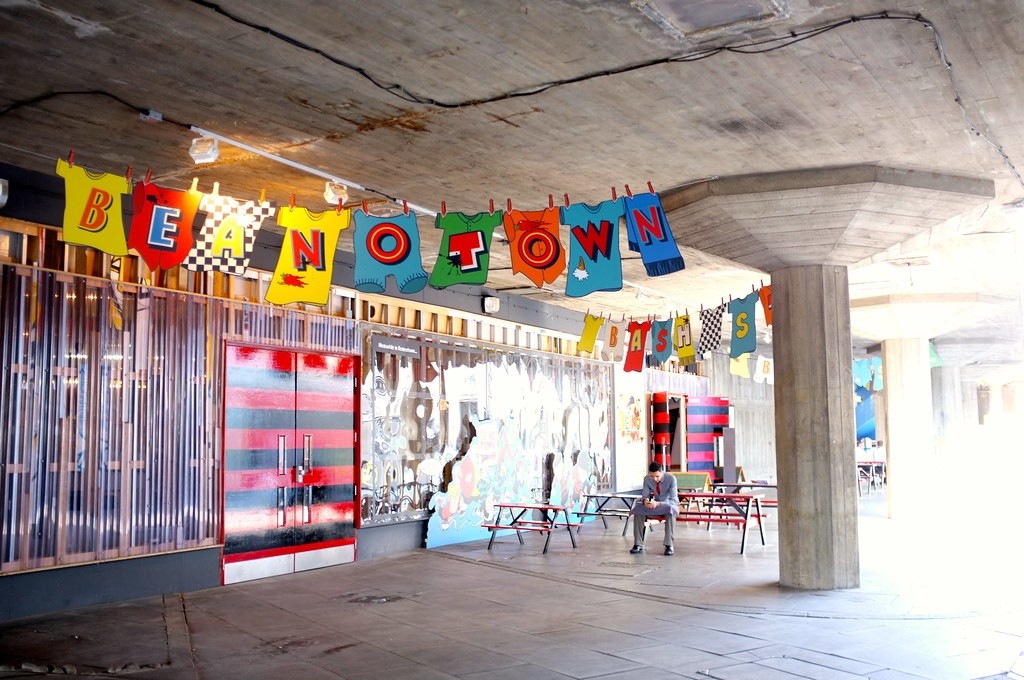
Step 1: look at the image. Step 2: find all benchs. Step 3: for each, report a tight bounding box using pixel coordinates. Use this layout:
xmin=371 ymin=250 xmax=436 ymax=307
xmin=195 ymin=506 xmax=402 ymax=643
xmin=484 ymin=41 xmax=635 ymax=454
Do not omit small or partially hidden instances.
xmin=481 ymin=499 xmax=779 ymax=553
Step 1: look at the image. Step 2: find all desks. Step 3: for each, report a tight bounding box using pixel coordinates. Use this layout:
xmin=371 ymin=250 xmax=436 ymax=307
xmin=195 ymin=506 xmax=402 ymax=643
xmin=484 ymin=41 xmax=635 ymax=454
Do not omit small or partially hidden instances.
xmin=487 ymin=461 xmax=887 ymax=554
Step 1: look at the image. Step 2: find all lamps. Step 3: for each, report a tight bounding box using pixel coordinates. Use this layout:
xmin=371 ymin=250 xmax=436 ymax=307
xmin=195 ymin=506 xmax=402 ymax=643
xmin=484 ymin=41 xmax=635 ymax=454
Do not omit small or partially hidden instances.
xmin=189 ymin=133 xmax=221 ymax=165
xmin=324 ymin=181 xmax=349 ymax=206
xmin=481 ymin=296 xmax=499 ymax=314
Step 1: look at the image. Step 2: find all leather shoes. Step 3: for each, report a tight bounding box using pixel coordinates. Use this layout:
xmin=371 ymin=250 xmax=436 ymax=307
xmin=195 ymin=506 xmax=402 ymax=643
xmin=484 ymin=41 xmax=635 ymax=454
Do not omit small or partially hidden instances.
xmin=630 ymin=544 xmax=643 ymax=554
xmin=663 ymin=547 xmax=675 ymax=556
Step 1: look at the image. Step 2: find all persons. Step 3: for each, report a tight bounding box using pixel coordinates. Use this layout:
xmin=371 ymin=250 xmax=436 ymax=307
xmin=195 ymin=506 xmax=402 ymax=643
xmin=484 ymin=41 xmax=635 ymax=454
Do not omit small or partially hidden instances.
xmin=629 ymin=461 xmax=680 ymax=556
xmin=856 ymin=439 xmax=886 ymax=485
